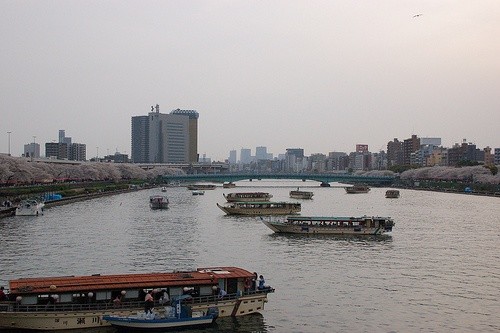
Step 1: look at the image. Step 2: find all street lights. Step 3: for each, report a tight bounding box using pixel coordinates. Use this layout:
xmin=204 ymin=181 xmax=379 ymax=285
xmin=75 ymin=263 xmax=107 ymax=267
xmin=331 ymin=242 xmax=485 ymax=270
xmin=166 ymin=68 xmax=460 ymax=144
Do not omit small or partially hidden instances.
xmin=96 ymin=147 xmax=98 ymax=161
xmin=33 ymin=136 xmax=36 ymax=157
xmin=8 ymin=131 xmax=12 ymax=154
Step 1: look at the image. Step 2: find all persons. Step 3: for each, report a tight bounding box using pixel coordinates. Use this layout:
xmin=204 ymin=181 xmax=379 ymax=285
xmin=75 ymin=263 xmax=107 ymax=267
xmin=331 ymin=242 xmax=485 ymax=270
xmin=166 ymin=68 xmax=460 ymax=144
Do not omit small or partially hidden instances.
xmin=257 ymin=274 xmax=265 ymax=293
xmin=315 ymin=222 xmax=337 ymax=225
xmin=145 ymin=291 xmax=154 ymax=315
xmin=159 ymin=289 xmax=169 ymax=300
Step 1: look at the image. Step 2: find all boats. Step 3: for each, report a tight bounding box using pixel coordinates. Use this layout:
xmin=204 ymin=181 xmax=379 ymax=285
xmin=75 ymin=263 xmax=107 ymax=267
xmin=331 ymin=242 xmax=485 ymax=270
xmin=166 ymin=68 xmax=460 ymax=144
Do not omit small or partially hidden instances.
xmin=0 ymin=267 xmax=275 ymax=331
xmin=103 ymin=303 xmax=219 ymax=331
xmin=149 ymin=196 xmax=169 ymax=209
xmin=385 ymin=190 xmax=400 ymax=198
xmin=16 ymin=199 xmax=45 ymax=216
xmin=344 ymin=182 xmax=371 ymax=193
xmin=320 ymin=181 xmax=330 ymax=187
xmin=259 ymin=215 xmax=395 ymax=234
xmin=217 ymin=202 xmax=301 ymax=215
xmin=222 ymin=192 xmax=273 ymax=202
xmin=161 ymin=186 xmax=167 ymax=192
xmin=289 ymin=187 xmax=314 ymax=200
xmin=188 ymin=182 xmax=235 ymax=195
